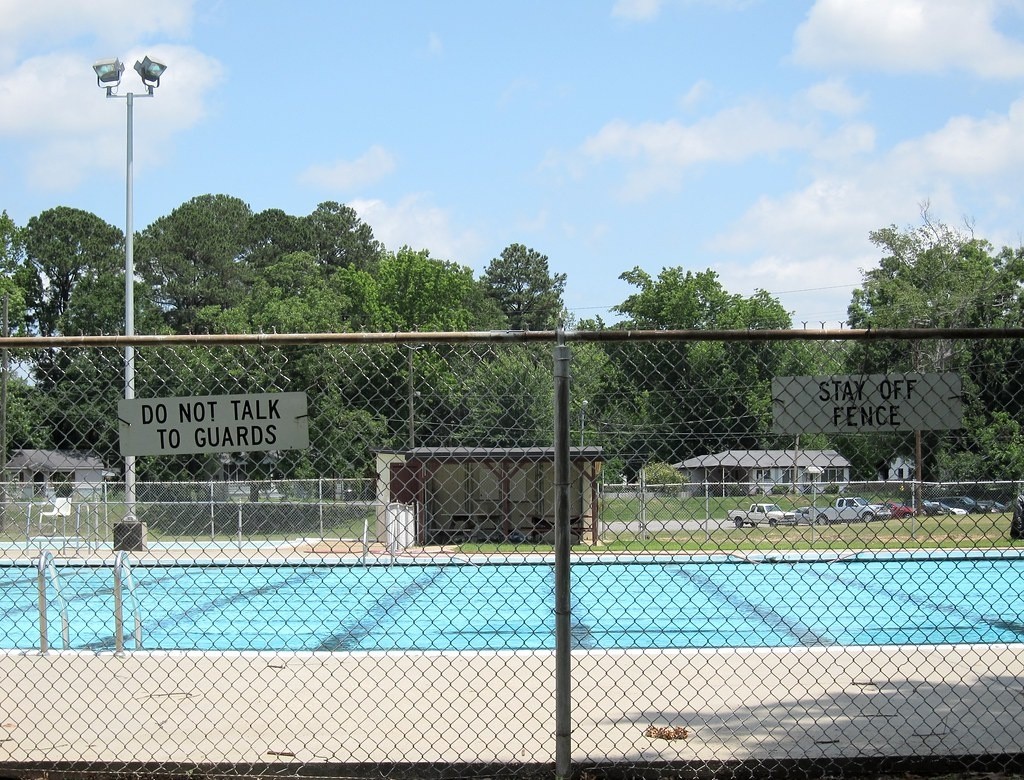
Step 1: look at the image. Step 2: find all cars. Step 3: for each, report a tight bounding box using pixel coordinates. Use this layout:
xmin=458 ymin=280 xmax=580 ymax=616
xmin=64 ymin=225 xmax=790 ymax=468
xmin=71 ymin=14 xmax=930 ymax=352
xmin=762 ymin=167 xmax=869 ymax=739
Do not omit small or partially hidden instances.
xmin=786 ymin=506 xmax=812 ymax=524
xmin=874 ymin=501 xmax=914 ymax=519
xmin=933 ymin=501 xmax=967 ymax=516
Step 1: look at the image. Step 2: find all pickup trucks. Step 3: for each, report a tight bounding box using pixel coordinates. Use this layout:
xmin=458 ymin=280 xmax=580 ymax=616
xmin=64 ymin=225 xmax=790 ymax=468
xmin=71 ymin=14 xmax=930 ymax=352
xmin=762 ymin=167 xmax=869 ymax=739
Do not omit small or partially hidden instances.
xmin=816 ymin=498 xmax=889 ymax=524
xmin=728 ymin=503 xmax=795 ymax=528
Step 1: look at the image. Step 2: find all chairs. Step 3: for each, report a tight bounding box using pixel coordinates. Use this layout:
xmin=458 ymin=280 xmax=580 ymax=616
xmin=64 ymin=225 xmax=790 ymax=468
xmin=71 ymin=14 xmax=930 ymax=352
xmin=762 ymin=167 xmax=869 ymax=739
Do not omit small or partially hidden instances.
xmin=37 ymin=498 xmax=71 ymax=555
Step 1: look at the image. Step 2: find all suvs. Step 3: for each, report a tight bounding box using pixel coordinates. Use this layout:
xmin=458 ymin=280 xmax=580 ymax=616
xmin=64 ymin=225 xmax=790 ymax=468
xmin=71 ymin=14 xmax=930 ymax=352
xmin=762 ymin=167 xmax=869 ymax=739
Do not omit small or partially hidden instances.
xmin=908 ymin=498 xmax=950 ymax=515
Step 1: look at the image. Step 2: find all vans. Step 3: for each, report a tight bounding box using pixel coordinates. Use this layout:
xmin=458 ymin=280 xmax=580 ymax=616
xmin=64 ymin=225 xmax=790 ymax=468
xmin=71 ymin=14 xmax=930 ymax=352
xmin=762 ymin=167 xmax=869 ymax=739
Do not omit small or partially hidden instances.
xmin=942 ymin=497 xmax=984 ymax=513
xmin=981 ymin=501 xmax=1007 ymax=513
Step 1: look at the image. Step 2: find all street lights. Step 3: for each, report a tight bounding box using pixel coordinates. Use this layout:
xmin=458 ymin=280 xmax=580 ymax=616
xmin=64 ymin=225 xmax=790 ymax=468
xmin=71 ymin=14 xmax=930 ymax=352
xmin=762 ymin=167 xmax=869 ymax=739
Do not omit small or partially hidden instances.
xmin=92 ymin=53 xmax=168 ymax=554
